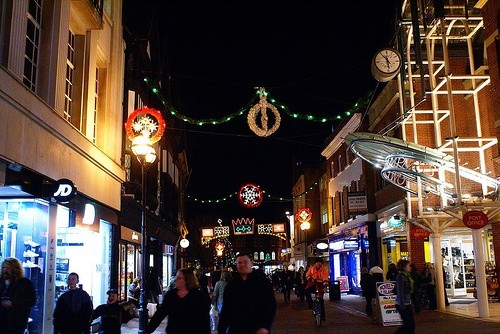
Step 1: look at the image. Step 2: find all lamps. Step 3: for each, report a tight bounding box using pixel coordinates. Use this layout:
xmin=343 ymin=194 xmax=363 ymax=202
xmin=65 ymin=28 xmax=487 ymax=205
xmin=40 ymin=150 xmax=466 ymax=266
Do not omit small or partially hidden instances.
xmin=180 ymin=237 xmax=189 ymax=248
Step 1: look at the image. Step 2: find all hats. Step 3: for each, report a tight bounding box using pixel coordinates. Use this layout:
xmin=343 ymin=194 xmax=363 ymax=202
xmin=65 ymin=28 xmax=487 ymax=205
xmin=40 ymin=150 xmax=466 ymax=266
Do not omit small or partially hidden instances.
xmin=315 ymin=257 xmax=323 ymax=263
xmin=107 ymin=289 xmax=118 ymax=294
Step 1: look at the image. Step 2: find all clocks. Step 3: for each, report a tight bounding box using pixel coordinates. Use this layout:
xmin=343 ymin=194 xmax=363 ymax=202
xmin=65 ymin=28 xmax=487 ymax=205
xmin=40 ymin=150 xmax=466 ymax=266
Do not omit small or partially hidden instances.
xmin=372 ymin=48 xmax=401 ymax=84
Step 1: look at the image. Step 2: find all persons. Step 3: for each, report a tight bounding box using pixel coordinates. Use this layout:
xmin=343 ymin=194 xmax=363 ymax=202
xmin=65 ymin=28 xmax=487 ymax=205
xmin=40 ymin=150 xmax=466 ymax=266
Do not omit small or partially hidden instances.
xmin=0 ymin=257 xmax=36 ymax=334
xmin=194 ymin=264 xmax=236 ymax=330
xmin=216 ymin=253 xmax=277 ymax=334
xmin=53 ymin=272 xmax=93 ymax=334
xmin=148 ymin=268 xmax=212 ymax=334
xmin=91 ymin=288 xmax=135 ymax=334
xmin=271 ymin=257 xmax=329 ymax=322
xmin=128 ymin=266 xmax=163 ymax=311
xmin=360 ymin=258 xmax=449 ymax=334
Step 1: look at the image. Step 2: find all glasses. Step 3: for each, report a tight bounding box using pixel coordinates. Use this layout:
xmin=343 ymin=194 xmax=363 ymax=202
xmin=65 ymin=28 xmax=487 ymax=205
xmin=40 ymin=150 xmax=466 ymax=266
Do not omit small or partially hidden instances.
xmin=176 ymin=276 xmax=184 ymax=280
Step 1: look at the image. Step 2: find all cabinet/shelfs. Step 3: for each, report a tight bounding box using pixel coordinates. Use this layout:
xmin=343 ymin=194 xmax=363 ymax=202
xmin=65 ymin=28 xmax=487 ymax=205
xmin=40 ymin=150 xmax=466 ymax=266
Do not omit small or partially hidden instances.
xmin=464 ymin=259 xmax=475 ymax=289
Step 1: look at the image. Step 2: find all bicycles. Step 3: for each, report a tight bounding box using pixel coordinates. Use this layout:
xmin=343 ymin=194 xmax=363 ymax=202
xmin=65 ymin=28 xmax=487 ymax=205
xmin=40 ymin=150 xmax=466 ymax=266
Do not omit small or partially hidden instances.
xmin=309 ymin=279 xmax=326 ymax=327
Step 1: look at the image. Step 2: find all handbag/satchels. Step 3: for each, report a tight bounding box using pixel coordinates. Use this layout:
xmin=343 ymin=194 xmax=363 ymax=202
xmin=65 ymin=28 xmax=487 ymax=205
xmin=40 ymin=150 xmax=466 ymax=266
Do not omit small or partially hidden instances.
xmin=393 ymin=285 xmax=397 ymax=295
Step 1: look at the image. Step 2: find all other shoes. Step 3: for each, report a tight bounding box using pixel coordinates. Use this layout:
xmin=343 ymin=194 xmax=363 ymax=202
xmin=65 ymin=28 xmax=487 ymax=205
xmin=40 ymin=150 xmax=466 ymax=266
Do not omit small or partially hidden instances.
xmin=321 ymin=315 xmax=326 ymax=321
xmin=308 ymin=302 xmax=312 ymax=307
xmin=367 ymin=311 xmax=373 ymax=315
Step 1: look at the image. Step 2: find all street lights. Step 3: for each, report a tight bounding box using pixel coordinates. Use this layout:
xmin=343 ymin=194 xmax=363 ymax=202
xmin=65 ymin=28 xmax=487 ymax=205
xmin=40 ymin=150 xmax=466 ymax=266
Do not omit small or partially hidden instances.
xmin=130 ymin=134 xmax=157 ymax=334
xmin=300 ymin=221 xmax=311 ymax=267
